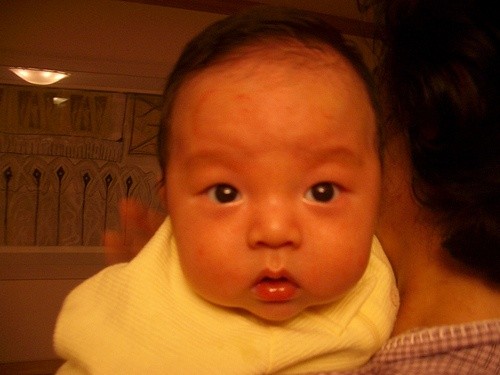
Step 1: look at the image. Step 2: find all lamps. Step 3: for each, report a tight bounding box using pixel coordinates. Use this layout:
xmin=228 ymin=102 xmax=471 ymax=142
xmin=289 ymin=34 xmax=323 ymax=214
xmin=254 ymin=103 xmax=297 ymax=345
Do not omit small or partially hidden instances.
xmin=7 ymin=66 xmax=71 ymax=85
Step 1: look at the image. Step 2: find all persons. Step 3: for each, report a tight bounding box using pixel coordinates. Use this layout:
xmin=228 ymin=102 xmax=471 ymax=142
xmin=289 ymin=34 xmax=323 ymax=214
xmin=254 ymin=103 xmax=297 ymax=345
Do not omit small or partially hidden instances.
xmin=49 ymin=7 xmax=399 ymax=374
xmin=94 ymin=1 xmax=500 ymax=375
